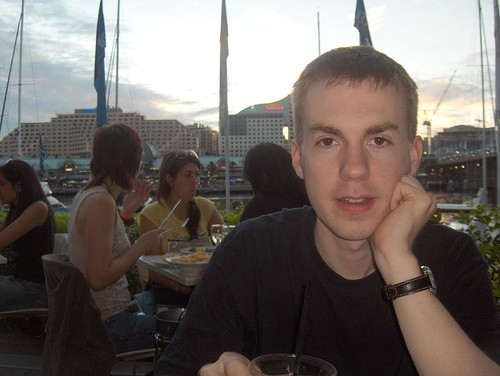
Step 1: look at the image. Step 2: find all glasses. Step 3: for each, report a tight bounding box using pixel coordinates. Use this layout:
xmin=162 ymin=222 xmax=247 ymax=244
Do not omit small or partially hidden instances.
xmin=5 ymin=159 xmax=20 ymax=179
xmin=172 ymin=149 xmax=199 ymax=165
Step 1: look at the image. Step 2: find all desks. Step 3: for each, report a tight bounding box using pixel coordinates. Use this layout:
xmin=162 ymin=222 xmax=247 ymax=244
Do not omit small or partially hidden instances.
xmin=138 ymin=256 xmax=201 ymax=297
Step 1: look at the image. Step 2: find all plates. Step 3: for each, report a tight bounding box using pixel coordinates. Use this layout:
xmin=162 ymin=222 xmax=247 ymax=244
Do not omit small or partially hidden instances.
xmin=180 ymin=246 xmax=218 ymax=254
xmin=166 ymin=255 xmax=211 ymax=264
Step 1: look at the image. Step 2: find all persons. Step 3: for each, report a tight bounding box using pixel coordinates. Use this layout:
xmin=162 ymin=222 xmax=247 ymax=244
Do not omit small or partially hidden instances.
xmin=67 ymin=123 xmax=155 ymax=352
xmin=0 ymin=159 xmax=55 ymax=307
xmin=155 ymin=47 xmax=500 ymax=376
xmin=138 ymin=151 xmax=224 ymax=306
xmin=240 ymin=144 xmax=312 ymax=221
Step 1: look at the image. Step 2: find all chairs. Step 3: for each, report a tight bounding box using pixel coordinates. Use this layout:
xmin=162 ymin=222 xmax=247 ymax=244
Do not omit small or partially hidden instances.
xmin=39 ymin=254 xmax=163 ymax=376
xmin=0 ymin=233 xmax=68 ymax=356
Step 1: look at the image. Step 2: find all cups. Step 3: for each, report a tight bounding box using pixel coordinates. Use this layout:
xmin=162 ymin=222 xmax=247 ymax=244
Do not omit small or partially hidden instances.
xmin=248 ymin=354 xmax=337 ymax=376
xmin=210 ymin=224 xmax=236 ymax=245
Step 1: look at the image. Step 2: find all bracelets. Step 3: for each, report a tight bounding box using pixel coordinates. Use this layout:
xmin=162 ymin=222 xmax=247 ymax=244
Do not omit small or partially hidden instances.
xmin=118 ymin=208 xmax=135 ymax=227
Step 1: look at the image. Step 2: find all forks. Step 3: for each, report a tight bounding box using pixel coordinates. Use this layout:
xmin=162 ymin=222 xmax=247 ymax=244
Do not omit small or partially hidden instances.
xmin=160 ymin=218 xmax=189 ymax=235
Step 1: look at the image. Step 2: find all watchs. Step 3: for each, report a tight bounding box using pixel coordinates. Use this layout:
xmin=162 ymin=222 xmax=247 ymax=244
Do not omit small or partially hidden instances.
xmin=381 ymin=265 xmax=437 ymax=300
